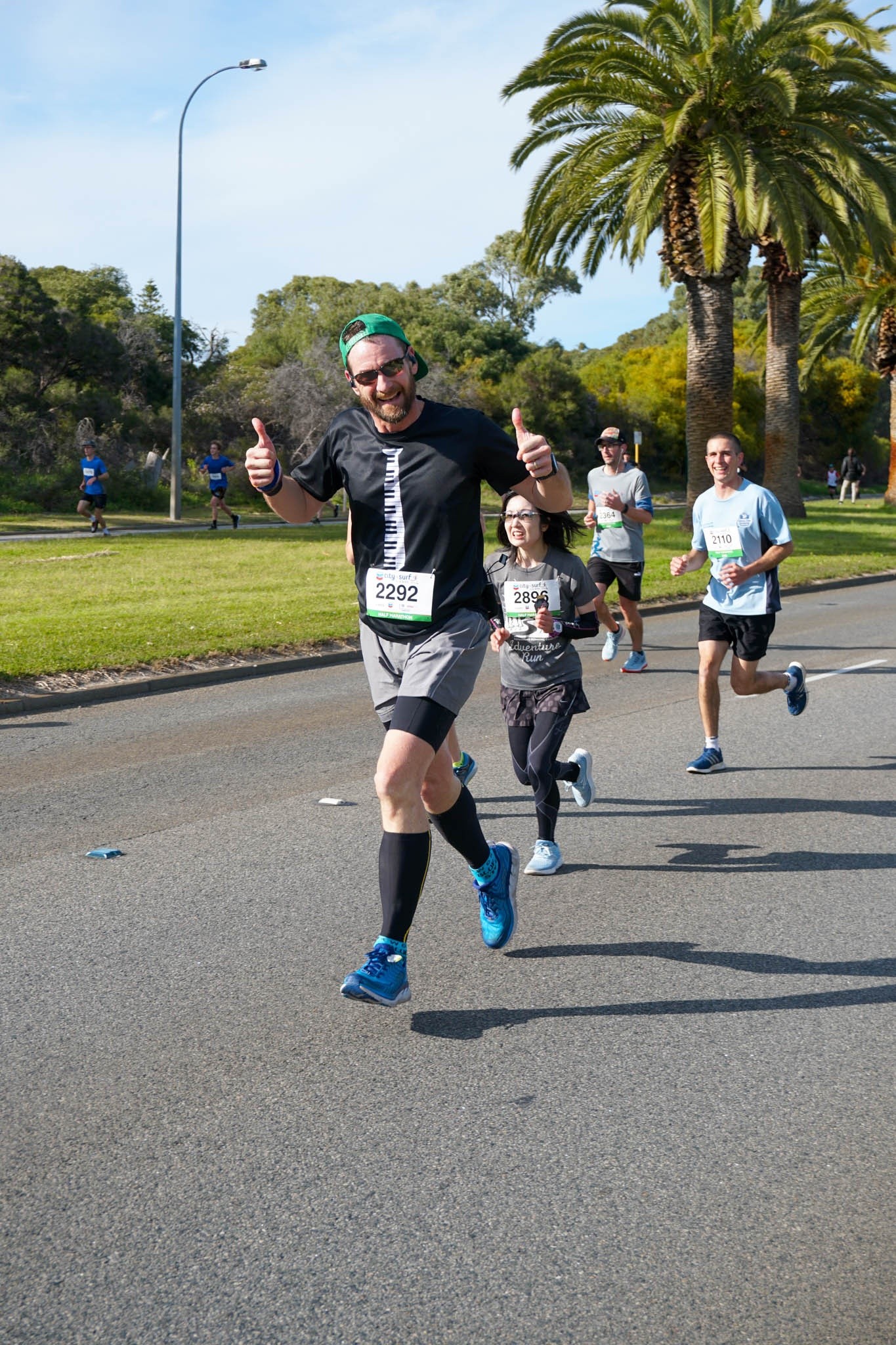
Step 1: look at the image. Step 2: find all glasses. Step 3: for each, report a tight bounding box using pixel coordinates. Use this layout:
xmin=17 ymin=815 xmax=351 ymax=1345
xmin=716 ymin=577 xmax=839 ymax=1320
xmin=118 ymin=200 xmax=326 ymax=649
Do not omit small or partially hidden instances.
xmin=348 ymin=352 xmax=409 ymax=386
xmin=499 ymin=510 xmax=542 ymax=522
xmin=597 ymin=443 xmax=621 ymax=451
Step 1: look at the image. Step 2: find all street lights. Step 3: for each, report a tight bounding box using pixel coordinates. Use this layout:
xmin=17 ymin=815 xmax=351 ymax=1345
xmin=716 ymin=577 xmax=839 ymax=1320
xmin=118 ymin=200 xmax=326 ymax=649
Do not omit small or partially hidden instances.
xmin=169 ymin=57 xmax=268 ymax=522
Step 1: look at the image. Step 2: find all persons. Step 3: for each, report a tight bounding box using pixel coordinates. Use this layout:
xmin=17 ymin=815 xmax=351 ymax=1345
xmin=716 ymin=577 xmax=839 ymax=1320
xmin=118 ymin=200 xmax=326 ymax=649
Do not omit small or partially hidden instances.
xmin=584 ymin=427 xmax=654 ymax=673
xmin=199 ymin=440 xmax=241 ymax=531
xmin=825 ymin=464 xmax=841 ymax=500
xmin=482 ymin=492 xmax=601 ymax=876
xmin=346 ymin=505 xmax=478 ymax=824
xmin=837 ymin=448 xmax=862 ymax=505
xmin=855 ymin=464 xmax=866 ymax=498
xmin=669 ymin=428 xmax=810 ymax=774
xmin=76 ymin=441 xmax=111 ymax=536
xmin=244 ymin=312 xmax=575 ymax=1008
xmin=311 ymin=501 xmax=340 ymax=525
xmin=797 ymin=466 xmax=802 ymax=477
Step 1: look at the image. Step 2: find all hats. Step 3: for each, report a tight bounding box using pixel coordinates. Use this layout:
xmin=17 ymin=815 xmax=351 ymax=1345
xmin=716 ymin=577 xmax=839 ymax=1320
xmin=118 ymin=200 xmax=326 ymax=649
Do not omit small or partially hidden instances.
xmin=340 ymin=314 xmax=430 ymax=385
xmin=595 ymin=427 xmax=625 ymax=447
xmin=81 ymin=441 xmax=95 ymax=447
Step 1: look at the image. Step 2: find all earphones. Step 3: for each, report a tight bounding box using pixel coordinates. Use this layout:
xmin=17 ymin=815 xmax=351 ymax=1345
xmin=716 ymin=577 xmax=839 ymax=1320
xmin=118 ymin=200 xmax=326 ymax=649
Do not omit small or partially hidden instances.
xmin=350 ymin=380 xmax=355 ymax=388
xmin=409 ymin=356 xmax=416 ymax=364
xmin=621 ymin=448 xmax=623 ymax=453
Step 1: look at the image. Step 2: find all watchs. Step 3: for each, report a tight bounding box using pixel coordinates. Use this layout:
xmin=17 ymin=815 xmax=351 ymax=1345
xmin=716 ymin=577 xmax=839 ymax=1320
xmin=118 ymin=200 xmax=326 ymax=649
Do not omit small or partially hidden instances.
xmin=549 ymin=620 xmax=563 ymax=638
xmin=534 ymin=453 xmax=559 ymax=483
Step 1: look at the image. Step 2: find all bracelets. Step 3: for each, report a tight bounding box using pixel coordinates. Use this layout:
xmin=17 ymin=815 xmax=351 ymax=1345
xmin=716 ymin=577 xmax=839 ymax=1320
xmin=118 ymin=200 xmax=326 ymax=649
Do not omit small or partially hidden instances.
xmin=621 ymin=503 xmax=629 ymax=514
xmin=256 ymin=459 xmax=283 ymax=496
xmin=96 ymin=476 xmax=98 ymax=480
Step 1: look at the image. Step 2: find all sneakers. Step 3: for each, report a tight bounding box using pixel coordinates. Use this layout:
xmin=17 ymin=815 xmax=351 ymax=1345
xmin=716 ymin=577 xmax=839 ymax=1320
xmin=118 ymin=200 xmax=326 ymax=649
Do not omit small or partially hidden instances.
xmin=310 ymin=518 xmax=320 ymax=525
xmin=619 ymin=652 xmax=648 ymax=673
xmin=564 ymin=747 xmax=595 ymax=807
xmin=472 ymin=841 xmax=521 ymax=950
xmin=91 ymin=520 xmax=99 ymax=532
xmin=686 ymin=747 xmax=727 ymax=774
xmin=525 ymin=838 xmax=563 ymax=876
xmin=602 ymin=620 xmax=626 ymax=662
xmin=232 ymin=514 xmax=240 ymax=529
xmin=206 ymin=524 xmax=218 ymax=531
xmin=453 ymin=751 xmax=478 ymax=788
xmin=783 ymin=662 xmax=808 ymax=716
xmin=340 ymin=945 xmax=413 ymax=1009
xmin=333 ymin=504 xmax=340 ymax=518
